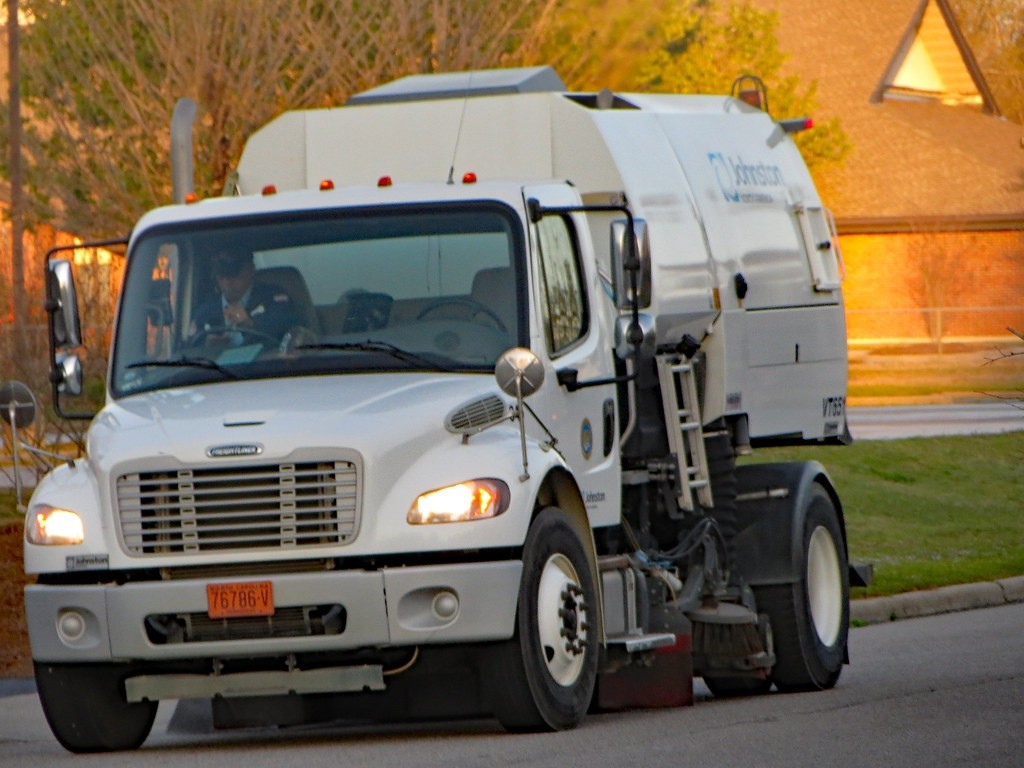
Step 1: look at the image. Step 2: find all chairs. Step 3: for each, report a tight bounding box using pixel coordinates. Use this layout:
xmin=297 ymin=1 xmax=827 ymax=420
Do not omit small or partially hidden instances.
xmin=470 ymin=267 xmax=518 ymax=350
xmin=237 ymin=268 xmax=317 ymax=348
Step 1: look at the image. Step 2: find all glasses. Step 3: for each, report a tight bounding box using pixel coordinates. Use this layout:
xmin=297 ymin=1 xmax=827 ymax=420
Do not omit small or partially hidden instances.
xmin=212 ymin=264 xmax=251 ymax=278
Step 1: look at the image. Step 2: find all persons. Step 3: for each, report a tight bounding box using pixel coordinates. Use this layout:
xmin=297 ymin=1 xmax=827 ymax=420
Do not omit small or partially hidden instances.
xmin=177 ymin=234 xmax=296 ymax=345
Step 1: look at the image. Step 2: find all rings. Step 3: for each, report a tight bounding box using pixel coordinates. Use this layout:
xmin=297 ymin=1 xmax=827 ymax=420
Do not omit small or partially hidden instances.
xmin=236 ymin=313 xmax=240 ymax=319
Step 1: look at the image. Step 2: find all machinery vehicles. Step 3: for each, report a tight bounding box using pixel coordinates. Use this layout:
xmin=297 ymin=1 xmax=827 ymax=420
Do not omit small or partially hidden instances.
xmin=1 ymin=67 xmax=856 ymax=755
